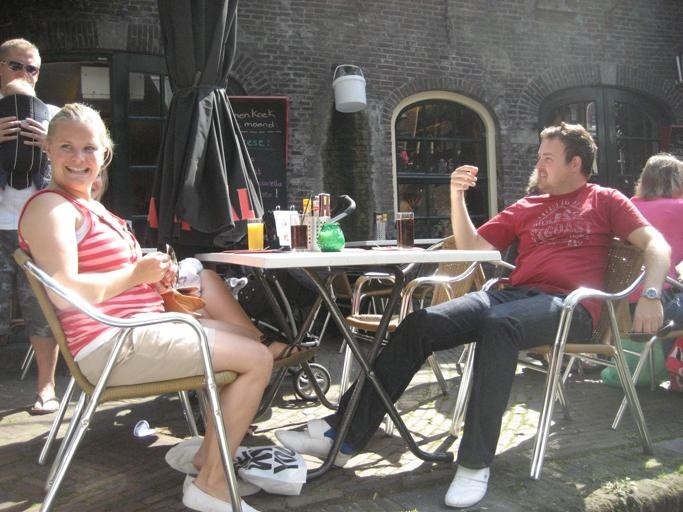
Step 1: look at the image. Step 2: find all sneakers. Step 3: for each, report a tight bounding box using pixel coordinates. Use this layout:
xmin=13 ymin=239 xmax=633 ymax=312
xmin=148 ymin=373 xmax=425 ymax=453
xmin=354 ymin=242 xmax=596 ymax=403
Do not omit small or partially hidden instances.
xmin=444 ymin=464 xmax=490 ymax=508
xmin=274 ymin=419 xmax=352 ymax=468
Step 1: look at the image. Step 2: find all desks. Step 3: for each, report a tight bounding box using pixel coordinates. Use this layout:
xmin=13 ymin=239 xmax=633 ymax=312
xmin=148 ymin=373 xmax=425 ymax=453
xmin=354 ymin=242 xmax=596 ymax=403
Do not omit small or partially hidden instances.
xmin=186 ymin=237 xmax=502 ymax=483
xmin=345 ymin=235 xmax=445 ymax=246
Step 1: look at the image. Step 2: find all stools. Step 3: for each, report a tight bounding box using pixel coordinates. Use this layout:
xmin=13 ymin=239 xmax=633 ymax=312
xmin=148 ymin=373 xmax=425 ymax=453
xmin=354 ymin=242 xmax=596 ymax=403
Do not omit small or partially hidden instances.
xmin=295 ymin=262 xmax=396 ymax=350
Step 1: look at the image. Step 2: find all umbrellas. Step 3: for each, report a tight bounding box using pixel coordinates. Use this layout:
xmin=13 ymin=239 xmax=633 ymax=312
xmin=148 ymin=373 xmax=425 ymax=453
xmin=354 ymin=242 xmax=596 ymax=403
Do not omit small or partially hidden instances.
xmin=149 ymin=0 xmax=265 ymax=250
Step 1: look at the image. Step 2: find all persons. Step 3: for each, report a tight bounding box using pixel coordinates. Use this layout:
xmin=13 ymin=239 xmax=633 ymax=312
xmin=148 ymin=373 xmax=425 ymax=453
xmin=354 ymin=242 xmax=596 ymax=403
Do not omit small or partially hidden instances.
xmin=0 ymin=37 xmax=64 ymax=414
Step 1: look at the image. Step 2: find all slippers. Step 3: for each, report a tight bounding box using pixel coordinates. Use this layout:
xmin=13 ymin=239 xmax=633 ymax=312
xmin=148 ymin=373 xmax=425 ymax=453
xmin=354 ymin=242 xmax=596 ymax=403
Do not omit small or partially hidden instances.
xmin=28 ymin=393 xmax=60 ymax=414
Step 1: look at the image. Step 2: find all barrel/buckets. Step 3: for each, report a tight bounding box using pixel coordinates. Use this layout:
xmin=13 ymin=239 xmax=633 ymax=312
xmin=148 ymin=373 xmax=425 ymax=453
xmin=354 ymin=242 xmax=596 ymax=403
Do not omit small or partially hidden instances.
xmin=332 ymin=63 xmax=367 ymax=114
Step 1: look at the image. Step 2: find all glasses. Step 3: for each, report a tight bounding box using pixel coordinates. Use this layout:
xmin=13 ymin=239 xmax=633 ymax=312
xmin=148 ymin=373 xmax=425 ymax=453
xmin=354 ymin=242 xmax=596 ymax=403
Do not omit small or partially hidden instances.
xmin=0 ymin=60 xmax=39 ymax=76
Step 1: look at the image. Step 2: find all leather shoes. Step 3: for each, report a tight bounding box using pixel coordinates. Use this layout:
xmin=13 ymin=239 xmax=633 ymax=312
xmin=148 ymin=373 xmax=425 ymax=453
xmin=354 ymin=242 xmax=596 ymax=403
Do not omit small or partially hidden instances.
xmin=182 ymin=474 xmax=262 ymax=512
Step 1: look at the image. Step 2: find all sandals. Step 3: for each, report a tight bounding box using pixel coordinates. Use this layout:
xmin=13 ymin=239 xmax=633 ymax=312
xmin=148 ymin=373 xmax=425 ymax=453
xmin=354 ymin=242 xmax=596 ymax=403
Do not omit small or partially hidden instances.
xmin=260 ymin=335 xmax=314 ymax=369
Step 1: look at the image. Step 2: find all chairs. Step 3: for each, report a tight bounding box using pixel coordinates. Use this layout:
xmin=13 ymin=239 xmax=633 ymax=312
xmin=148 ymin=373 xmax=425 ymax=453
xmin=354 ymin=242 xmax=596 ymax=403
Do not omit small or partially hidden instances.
xmin=596 ymin=247 xmax=683 ymax=432
xmin=335 ymin=230 xmax=483 ymax=411
xmin=443 ymin=222 xmax=670 ymax=487
xmin=10 ymin=247 xmax=250 ymax=512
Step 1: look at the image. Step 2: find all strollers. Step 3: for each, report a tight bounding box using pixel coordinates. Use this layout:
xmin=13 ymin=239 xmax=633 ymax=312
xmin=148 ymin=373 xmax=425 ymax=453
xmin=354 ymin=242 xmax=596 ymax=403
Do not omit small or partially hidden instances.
xmin=206 ymin=265 xmax=333 ymax=419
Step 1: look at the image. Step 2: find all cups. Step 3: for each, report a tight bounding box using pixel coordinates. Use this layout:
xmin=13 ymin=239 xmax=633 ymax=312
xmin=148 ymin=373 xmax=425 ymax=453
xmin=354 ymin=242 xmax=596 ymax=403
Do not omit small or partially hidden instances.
xmin=394 ymin=212 xmax=415 ymax=249
xmin=290 ymin=214 xmax=308 ymax=251
xmin=246 ymin=217 xmax=264 ymax=251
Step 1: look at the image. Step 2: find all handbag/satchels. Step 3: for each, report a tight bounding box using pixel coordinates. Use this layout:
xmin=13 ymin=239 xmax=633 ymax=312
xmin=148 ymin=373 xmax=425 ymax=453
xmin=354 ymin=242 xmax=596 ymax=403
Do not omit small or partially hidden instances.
xmin=600 ymin=339 xmax=665 ymax=387
xmin=165 ymin=439 xmax=307 ymax=496
xmin=160 ymin=286 xmax=206 ymax=320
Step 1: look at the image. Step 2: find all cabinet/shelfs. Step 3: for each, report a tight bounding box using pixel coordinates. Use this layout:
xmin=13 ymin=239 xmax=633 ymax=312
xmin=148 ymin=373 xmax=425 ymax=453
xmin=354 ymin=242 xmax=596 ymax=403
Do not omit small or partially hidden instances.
xmin=392 ymin=131 xmax=487 ymax=225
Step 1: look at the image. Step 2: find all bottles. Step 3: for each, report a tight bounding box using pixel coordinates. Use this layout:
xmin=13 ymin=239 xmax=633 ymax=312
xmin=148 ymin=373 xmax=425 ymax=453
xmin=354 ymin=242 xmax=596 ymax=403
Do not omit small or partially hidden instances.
xmin=399 ymin=140 xmax=437 ymax=173
xmin=301 ymin=199 xmax=319 ymax=251
xmin=316 ymin=223 xmax=345 ymax=251
